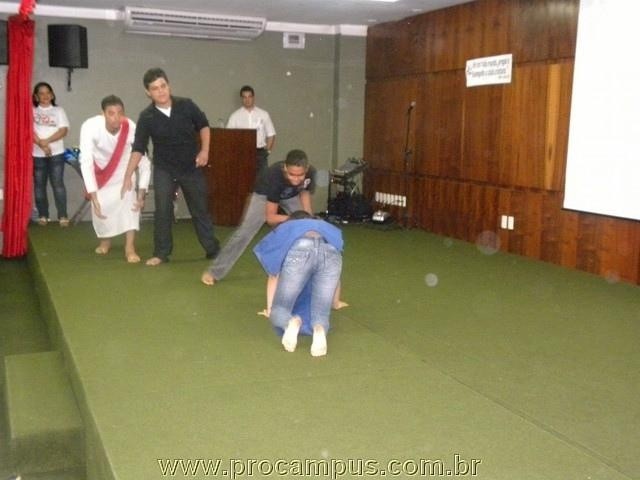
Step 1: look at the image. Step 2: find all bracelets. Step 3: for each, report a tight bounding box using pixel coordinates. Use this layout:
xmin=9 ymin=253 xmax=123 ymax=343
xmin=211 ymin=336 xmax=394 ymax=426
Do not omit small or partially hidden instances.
xmin=265 ymin=149 xmax=273 ymax=155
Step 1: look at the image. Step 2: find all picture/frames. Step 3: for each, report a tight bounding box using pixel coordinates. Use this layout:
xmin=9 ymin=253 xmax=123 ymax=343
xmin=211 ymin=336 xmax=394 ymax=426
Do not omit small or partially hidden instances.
xmin=282 ymin=30 xmax=308 ymax=49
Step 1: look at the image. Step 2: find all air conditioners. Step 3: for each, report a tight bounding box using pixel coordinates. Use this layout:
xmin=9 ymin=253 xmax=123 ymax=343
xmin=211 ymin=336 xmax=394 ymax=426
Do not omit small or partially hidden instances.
xmin=123 ymin=4 xmax=266 ymax=42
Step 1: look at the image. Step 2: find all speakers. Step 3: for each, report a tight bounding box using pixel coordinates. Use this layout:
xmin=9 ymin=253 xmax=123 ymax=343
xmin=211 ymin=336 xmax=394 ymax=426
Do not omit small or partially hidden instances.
xmin=0 ymin=20 xmax=9 ymax=65
xmin=48 ymin=24 xmax=87 ymax=68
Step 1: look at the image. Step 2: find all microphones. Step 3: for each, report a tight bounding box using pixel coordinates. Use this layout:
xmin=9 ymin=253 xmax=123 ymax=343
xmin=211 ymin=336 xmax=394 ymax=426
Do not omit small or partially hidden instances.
xmin=408 ymin=101 xmax=416 ymax=114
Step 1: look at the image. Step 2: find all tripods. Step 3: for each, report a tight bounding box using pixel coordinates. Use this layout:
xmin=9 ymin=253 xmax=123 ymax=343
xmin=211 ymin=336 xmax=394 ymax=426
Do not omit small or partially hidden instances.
xmin=385 ymin=114 xmax=431 ymax=232
xmin=322 ymin=180 xmax=359 ymax=225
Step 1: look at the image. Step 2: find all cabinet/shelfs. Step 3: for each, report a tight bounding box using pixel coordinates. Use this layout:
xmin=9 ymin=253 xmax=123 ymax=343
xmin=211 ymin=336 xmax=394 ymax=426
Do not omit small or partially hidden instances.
xmin=63 ymin=154 xmax=179 ymax=225
xmin=327 ymin=166 xmax=371 ymax=224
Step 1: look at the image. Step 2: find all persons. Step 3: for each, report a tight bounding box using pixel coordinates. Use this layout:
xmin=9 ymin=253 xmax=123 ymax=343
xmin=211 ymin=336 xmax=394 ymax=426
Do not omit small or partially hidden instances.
xmin=225 ymin=86 xmax=276 ymax=173
xmin=79 ymin=95 xmax=151 ymax=264
xmin=257 ymin=210 xmax=349 ymax=358
xmin=201 ymin=151 xmax=316 ymax=287
xmin=121 ymin=68 xmax=221 ymax=267
xmin=30 ymin=82 xmax=72 ymax=229
